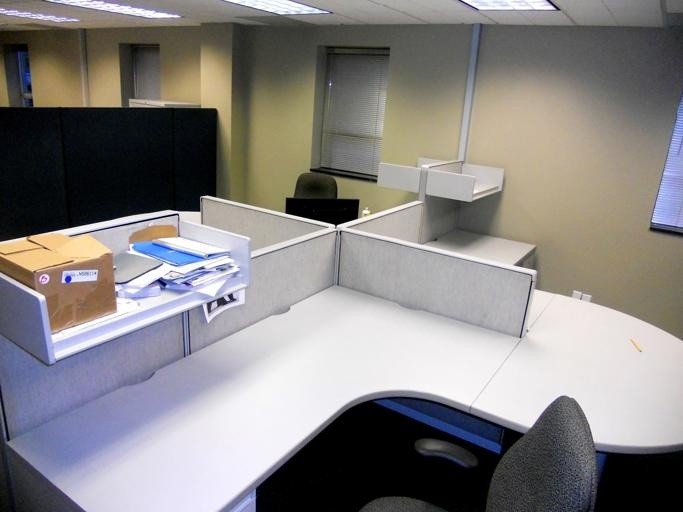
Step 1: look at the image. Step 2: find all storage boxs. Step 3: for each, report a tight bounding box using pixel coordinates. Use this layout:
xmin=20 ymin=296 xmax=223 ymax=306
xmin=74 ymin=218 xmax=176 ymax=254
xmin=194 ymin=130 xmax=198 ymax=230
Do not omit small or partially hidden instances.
xmin=2 ymin=232 xmax=118 ymax=334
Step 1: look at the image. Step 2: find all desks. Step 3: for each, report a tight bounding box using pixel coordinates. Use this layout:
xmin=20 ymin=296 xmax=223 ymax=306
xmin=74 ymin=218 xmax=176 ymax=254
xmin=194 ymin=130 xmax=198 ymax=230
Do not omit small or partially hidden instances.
xmin=3 ymin=159 xmax=682 ymax=509
xmin=418 ymin=227 xmax=539 ymax=272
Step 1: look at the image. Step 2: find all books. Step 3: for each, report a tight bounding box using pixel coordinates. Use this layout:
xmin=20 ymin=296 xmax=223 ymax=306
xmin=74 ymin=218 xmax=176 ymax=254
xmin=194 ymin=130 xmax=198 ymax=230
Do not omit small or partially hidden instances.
xmin=107 ymin=235 xmax=241 ymax=302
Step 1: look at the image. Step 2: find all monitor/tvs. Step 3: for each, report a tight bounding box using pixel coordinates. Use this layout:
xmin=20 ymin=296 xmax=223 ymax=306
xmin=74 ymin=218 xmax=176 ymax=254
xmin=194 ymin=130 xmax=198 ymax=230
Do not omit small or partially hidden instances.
xmin=285 ymin=197 xmax=360 ymax=225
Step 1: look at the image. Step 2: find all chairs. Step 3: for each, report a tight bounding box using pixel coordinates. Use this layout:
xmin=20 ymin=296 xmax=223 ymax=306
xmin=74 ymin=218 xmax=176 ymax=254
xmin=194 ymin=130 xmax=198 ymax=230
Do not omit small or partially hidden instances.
xmin=356 ymin=393 xmax=599 ymax=509
xmin=292 ymin=171 xmax=339 ymax=198
